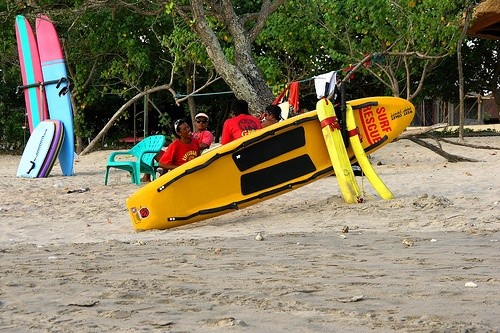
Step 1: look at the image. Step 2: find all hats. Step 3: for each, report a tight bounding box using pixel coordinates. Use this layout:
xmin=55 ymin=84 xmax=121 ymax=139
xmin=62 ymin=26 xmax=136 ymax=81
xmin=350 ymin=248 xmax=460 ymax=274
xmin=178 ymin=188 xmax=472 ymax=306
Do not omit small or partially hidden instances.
xmin=195 ymin=112 xmax=209 ymax=121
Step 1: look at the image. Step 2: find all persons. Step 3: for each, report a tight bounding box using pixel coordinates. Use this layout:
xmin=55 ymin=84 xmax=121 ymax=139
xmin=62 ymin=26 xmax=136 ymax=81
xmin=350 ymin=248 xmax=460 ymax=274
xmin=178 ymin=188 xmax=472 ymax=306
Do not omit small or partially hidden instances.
xmin=140 ymin=112 xmax=214 ymax=182
xmin=158 ymin=119 xmax=201 ymax=169
xmin=220 ymin=99 xmax=264 ymax=145
xmin=264 ymin=106 xmax=282 ymax=126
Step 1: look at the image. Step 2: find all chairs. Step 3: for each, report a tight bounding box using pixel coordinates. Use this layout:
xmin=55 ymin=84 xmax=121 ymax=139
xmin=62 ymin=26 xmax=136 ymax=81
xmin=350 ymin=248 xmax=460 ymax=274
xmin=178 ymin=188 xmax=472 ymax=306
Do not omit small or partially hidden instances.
xmin=104 ymin=133 xmax=167 ymax=185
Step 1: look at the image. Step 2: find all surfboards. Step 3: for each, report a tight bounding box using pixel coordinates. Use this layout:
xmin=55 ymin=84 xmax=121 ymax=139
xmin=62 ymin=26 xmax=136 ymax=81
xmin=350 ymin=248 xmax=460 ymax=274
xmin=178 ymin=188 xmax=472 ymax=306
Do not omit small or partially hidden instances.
xmin=36 ymin=12 xmax=74 ymax=175
xmin=16 ymin=120 xmax=55 ymax=179
xmin=126 ymin=96 xmax=415 ymax=230
xmin=14 ymin=14 xmax=48 ymax=134
xmin=38 ymin=120 xmax=65 ymax=178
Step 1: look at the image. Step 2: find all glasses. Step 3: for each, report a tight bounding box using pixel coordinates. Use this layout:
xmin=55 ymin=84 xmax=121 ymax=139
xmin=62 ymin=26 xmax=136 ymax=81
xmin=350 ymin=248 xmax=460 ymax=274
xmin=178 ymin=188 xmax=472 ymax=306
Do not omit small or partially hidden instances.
xmin=173 ymin=118 xmax=183 ymax=132
xmin=265 ymin=114 xmax=270 ymax=117
xmin=197 ymin=121 xmax=207 ymax=123
